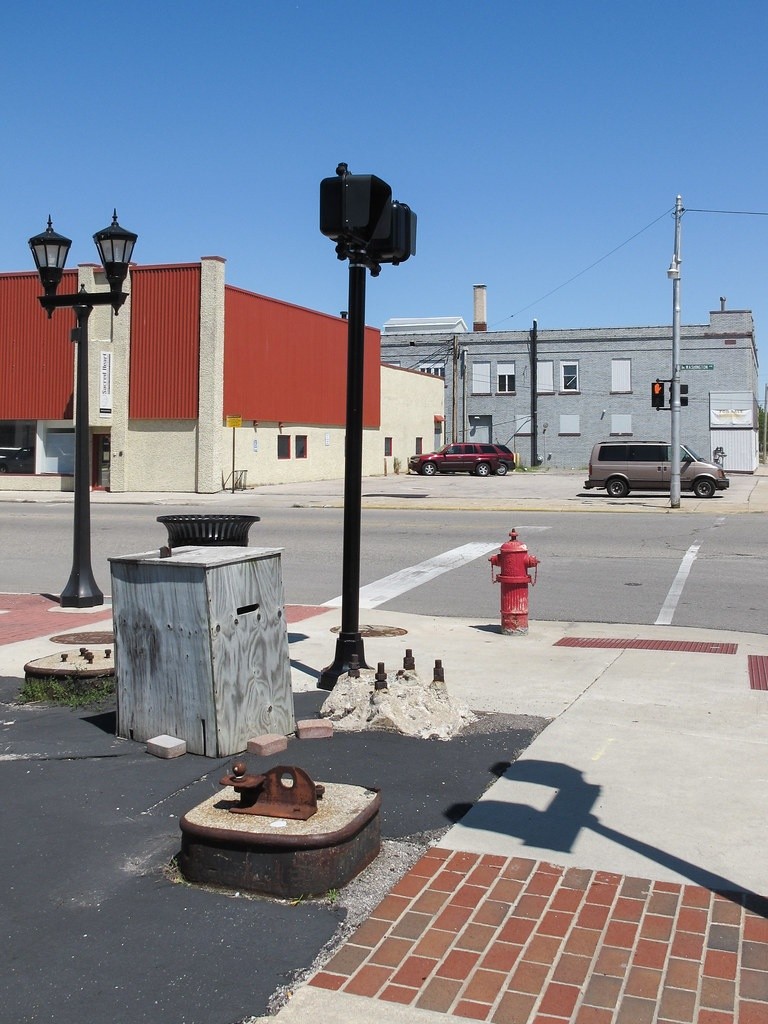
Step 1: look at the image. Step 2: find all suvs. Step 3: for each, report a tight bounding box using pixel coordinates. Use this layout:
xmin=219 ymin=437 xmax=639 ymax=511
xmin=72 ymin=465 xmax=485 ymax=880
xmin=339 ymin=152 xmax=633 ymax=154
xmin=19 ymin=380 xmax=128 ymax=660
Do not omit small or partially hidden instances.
xmin=408 ymin=442 xmax=516 ymax=475
xmin=583 ymin=440 xmax=730 ymax=497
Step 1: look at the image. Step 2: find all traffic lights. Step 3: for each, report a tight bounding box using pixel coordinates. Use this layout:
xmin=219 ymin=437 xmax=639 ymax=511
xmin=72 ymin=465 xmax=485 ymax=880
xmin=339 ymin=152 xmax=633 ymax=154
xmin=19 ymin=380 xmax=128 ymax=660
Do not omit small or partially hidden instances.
xmin=651 ymin=382 xmax=665 ymax=407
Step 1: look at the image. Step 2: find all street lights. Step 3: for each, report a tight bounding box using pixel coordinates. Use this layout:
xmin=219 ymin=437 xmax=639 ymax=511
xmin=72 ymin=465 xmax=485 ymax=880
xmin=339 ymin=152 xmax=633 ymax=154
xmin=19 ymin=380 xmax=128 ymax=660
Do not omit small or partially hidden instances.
xmin=668 ymin=253 xmax=681 ymax=509
xmin=25 ymin=207 xmax=138 ymax=607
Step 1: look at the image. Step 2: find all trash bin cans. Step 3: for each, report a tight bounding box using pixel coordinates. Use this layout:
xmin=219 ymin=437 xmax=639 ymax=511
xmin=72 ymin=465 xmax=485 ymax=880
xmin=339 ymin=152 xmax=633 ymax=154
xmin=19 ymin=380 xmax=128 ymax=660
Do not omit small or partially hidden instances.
xmin=156 ymin=514 xmax=261 ymax=548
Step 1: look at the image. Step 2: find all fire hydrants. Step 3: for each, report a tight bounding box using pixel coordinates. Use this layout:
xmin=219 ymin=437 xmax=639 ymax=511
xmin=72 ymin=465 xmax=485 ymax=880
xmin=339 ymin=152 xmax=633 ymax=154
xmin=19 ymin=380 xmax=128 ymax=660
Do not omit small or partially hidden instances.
xmin=487 ymin=527 xmax=540 ymax=637
